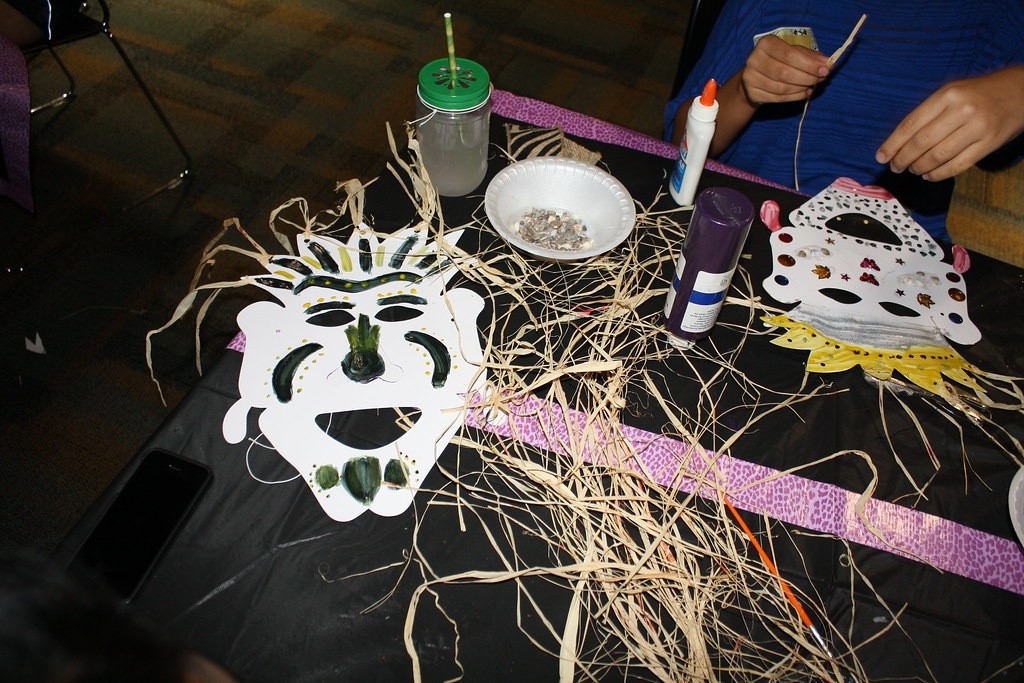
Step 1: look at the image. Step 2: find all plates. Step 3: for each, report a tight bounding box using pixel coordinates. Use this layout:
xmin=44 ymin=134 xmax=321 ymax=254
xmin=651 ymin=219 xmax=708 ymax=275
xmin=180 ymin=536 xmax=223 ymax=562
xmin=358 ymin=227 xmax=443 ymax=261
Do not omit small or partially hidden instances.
xmin=1009 ymin=466 xmax=1024 ymax=546
xmin=485 ymin=157 xmax=637 ymax=263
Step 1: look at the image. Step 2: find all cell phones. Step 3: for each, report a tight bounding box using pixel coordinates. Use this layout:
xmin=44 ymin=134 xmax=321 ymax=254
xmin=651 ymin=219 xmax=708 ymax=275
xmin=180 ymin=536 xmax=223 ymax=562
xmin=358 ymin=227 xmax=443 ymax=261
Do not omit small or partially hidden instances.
xmin=66 ymin=448 xmax=214 ymax=603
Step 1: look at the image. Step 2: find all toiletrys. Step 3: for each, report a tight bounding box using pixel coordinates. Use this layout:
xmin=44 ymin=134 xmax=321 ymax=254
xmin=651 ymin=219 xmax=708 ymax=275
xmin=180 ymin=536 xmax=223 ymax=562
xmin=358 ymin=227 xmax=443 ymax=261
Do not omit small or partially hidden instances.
xmin=664 ymin=186 xmax=755 ymax=340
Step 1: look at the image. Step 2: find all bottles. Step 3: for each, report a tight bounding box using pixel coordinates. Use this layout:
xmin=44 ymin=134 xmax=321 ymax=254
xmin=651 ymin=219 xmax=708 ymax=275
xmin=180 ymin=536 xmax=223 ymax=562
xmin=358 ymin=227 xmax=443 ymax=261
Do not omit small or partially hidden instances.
xmin=670 ymin=79 xmax=719 ymax=205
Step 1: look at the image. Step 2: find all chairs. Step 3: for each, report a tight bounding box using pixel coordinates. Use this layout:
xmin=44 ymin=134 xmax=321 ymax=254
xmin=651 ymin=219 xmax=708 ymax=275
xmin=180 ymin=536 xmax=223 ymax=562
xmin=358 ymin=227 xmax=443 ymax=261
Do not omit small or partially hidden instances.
xmin=0 ymin=0 xmax=193 ymax=289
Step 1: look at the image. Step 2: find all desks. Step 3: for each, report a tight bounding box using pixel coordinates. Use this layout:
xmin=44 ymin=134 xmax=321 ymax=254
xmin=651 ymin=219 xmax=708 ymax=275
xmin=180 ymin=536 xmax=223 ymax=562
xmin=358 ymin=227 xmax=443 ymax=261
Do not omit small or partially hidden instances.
xmin=51 ymin=84 xmax=1024 ymax=683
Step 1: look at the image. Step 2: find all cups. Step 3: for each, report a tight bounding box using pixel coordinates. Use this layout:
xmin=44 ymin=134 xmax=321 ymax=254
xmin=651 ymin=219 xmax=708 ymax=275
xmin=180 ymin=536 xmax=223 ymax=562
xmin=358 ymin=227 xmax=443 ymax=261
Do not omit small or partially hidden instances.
xmin=416 ymin=58 xmax=491 ymax=195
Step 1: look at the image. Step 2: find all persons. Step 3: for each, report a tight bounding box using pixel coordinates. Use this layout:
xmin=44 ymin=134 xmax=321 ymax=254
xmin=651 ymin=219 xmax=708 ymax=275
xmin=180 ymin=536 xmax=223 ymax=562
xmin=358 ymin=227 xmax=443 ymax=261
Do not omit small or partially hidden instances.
xmin=662 ymin=0 xmax=1024 ymax=257
